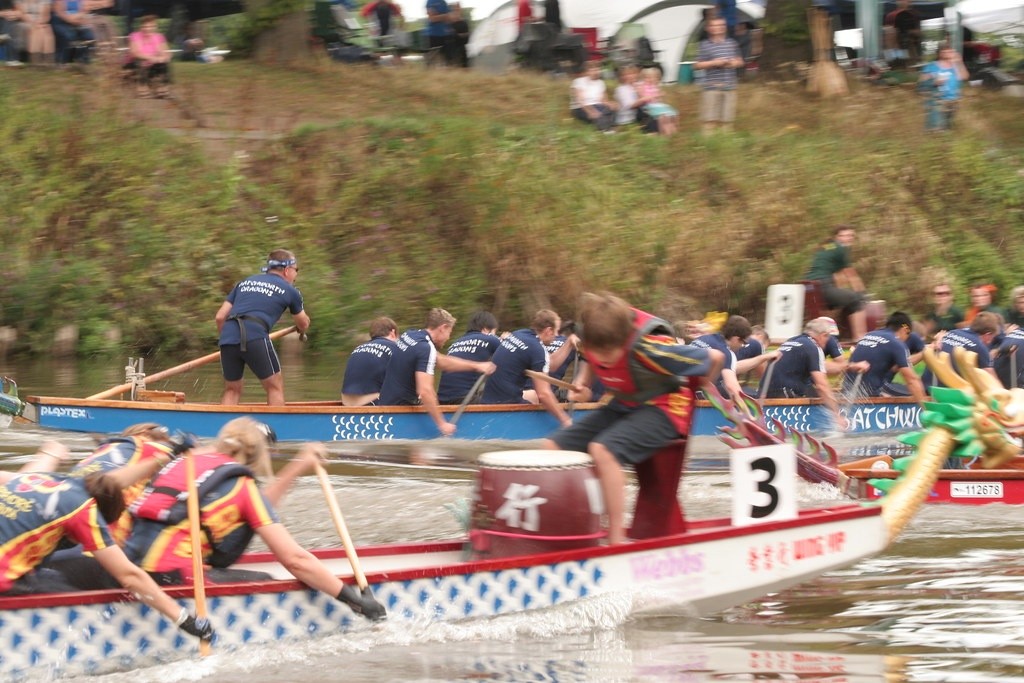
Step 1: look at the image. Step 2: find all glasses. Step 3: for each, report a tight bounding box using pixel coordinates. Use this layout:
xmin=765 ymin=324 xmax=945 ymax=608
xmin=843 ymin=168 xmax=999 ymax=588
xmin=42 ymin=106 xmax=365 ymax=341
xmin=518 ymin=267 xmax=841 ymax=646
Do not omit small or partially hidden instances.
xmin=739 ymin=338 xmax=751 ymax=349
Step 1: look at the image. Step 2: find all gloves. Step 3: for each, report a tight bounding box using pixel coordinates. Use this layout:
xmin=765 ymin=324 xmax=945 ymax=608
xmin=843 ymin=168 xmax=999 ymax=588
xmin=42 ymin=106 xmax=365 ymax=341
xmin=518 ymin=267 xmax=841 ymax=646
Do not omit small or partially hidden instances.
xmin=178 ymin=612 xmax=217 ymax=641
xmin=337 ymin=583 xmax=389 ymax=624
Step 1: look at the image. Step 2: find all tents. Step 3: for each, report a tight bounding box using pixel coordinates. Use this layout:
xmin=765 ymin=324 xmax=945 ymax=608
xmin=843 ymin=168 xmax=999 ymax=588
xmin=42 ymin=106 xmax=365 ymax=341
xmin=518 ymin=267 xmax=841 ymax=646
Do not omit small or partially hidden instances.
xmin=952 ymin=0 xmax=1024 ymax=45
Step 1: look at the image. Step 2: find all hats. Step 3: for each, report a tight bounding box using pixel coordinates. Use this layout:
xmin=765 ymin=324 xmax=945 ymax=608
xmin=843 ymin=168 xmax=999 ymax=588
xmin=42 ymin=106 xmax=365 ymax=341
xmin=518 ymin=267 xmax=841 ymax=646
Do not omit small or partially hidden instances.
xmin=820 ymin=317 xmax=839 ymax=335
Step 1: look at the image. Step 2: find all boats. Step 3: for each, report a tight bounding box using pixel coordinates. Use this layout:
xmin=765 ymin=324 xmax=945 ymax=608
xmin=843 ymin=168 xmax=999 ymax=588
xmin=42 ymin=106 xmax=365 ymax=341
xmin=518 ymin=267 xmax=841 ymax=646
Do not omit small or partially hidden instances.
xmin=0 ymin=338 xmax=1024 ymax=683
xmin=27 ymin=392 xmax=925 ymax=467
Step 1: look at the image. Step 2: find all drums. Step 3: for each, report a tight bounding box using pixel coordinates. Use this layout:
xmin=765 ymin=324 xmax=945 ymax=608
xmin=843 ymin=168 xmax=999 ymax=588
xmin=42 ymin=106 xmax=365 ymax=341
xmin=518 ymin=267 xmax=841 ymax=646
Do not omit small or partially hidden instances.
xmin=470 ymin=449 xmax=604 ymax=559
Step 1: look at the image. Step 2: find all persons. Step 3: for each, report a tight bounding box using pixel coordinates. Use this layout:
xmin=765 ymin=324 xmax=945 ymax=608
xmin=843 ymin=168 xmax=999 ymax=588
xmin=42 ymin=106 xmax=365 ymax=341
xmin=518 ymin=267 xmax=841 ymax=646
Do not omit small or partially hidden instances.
xmin=687 ymin=314 xmax=758 ymax=423
xmin=614 ymin=63 xmax=653 ymax=133
xmin=215 ymin=249 xmax=310 ymax=406
xmin=541 ymin=292 xmax=724 ymax=548
xmin=377 ymin=308 xmax=498 ymax=435
xmin=342 ymin=313 xmax=398 ymax=407
xmin=0 ymin=417 xmax=386 ymax=644
xmin=480 ymin=309 xmax=573 ymax=428
xmin=121 ymin=14 xmax=174 ymax=97
xmin=638 ymin=64 xmax=680 ymax=136
xmin=916 ymin=46 xmax=969 ymax=130
xmin=808 ymin=226 xmax=874 ymax=344
xmin=758 ymin=316 xmax=849 ymax=426
xmin=340 ymin=227 xmax=1024 ymax=414
xmin=569 ymin=59 xmax=618 ymax=131
xmin=693 ymin=15 xmax=745 ymax=135
xmin=0 ymin=0 xmax=238 ymax=100
xmin=341 ymin=0 xmax=1007 ymax=135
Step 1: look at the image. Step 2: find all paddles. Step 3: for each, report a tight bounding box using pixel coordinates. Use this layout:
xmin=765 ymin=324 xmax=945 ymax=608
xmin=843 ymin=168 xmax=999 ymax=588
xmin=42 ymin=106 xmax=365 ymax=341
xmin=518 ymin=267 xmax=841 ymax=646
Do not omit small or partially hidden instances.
xmin=758 ymin=363 xmax=776 ymax=409
xmin=185 ymin=447 xmax=213 ymax=657
xmin=1009 ymin=353 xmax=1018 ymax=387
xmin=846 ymin=374 xmax=864 ymax=416
xmin=567 ymin=352 xmax=581 ymax=417
xmin=314 ymin=465 xmax=374 ymax=598
xmin=85 ymin=325 xmax=298 ymax=400
xmin=931 ymin=348 xmax=939 ymax=403
xmin=448 ymin=373 xmax=487 ymax=425
